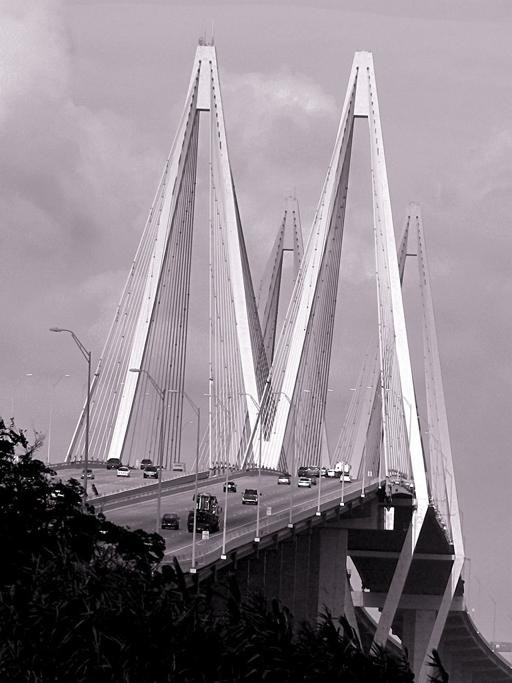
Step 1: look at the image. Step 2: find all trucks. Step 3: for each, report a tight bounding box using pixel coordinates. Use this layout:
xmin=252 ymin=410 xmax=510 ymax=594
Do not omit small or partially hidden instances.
xmin=172 ymin=463 xmax=184 ymax=471
xmin=187 ymin=494 xmax=220 ymax=532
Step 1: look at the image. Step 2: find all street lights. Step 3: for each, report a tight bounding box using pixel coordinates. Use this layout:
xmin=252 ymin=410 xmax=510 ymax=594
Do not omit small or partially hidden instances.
xmin=303 ymin=387 xmax=390 ymax=514
xmin=130 ymin=369 xmax=166 ymax=570
xmin=203 ymin=394 xmax=234 ymax=556
xmin=273 ymin=392 xmax=296 ymax=524
xmin=166 ymin=388 xmax=201 ymax=568
xmin=238 ymin=393 xmax=263 ymax=537
xmin=48 ymin=325 xmax=91 ymax=505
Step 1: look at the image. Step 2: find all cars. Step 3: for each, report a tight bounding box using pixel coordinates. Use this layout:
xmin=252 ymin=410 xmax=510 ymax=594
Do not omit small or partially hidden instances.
xmin=278 ymin=475 xmax=290 ymax=484
xmin=140 ymin=458 xmax=164 ymax=479
xmin=222 ymin=481 xmax=262 ymax=506
xmin=81 ymin=468 xmax=95 ymax=479
xmin=161 ymin=513 xmax=180 ymax=529
xmin=298 ymin=477 xmax=312 ymax=488
xmin=107 ymin=458 xmax=130 ymax=476
